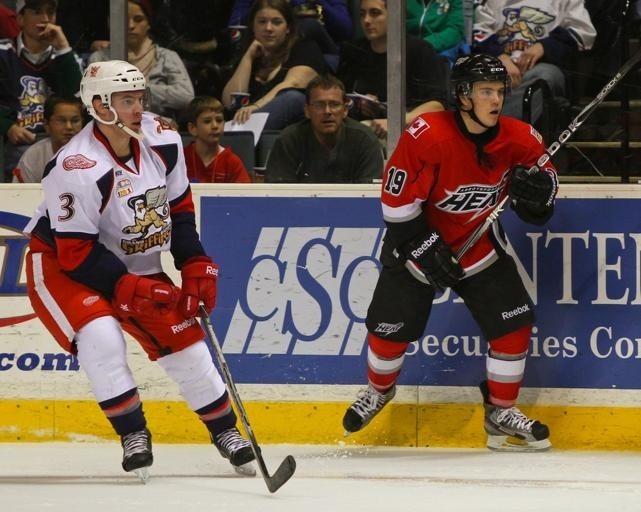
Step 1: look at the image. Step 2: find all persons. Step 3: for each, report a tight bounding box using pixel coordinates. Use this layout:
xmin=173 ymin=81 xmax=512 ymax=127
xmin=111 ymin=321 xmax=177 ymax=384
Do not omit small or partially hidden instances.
xmin=24 ymin=59 xmax=261 ymax=474
xmin=342 ymin=51 xmax=560 ymax=450
xmin=0 ymin=1 xmax=598 ymax=184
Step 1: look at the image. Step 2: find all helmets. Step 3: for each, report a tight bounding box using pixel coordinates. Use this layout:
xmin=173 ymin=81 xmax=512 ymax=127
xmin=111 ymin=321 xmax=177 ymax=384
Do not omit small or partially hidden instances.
xmin=80 ymin=60 xmax=146 ymax=125
xmin=450 ymin=54 xmax=508 ymax=100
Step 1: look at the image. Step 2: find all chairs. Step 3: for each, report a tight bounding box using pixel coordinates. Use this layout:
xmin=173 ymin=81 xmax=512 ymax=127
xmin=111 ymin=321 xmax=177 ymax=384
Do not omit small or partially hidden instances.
xmin=183 ymin=128 xmax=256 ymax=183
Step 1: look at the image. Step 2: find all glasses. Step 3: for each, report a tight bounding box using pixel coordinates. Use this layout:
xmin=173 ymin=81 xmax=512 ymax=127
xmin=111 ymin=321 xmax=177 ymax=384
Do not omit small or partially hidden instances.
xmin=310 ymin=101 xmax=345 ymax=109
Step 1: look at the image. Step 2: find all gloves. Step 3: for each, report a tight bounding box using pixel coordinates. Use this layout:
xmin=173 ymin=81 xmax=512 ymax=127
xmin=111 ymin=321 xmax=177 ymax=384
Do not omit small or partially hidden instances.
xmin=509 ymin=163 xmax=554 ymax=227
xmin=405 ymin=224 xmax=464 ymax=287
xmin=115 ymin=257 xmax=218 ymax=344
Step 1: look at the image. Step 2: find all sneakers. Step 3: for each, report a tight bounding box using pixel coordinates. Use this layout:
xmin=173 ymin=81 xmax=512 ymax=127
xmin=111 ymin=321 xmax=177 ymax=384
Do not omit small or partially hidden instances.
xmin=344 ymin=386 xmax=397 ymax=432
xmin=122 ymin=426 xmax=154 ymax=470
xmin=211 ymin=426 xmax=259 ymax=468
xmin=483 ymin=401 xmax=549 ymax=444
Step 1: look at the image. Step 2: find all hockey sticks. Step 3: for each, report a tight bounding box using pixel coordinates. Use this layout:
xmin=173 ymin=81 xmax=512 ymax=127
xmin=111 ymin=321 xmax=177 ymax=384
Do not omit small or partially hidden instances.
xmin=199 ymin=300 xmax=295 ymax=493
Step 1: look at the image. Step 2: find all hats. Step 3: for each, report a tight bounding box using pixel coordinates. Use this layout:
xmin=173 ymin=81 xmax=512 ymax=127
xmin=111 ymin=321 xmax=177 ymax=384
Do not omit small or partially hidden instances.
xmin=17 ymin=0 xmax=57 ymax=13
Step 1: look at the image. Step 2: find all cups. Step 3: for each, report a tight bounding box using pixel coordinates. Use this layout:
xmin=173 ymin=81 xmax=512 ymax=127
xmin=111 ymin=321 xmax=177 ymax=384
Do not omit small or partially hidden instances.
xmin=228 ymin=25 xmax=246 ymax=51
xmin=230 ymin=92 xmax=250 ymax=112
xmin=345 ymin=94 xmax=360 ymax=121
